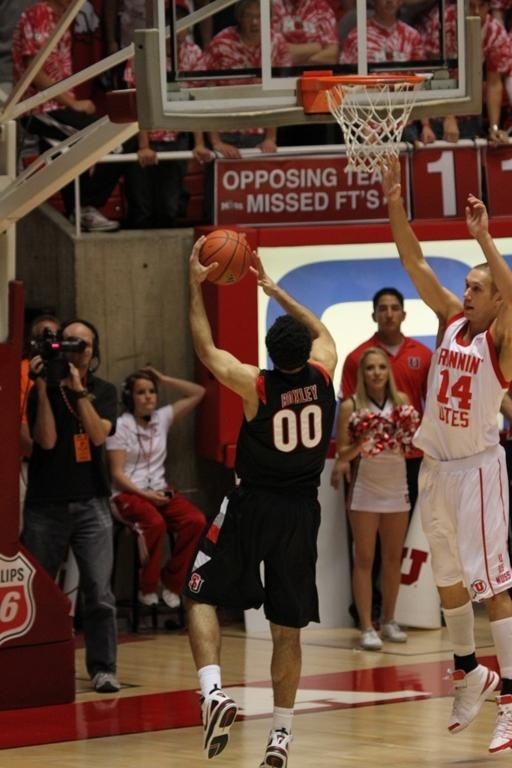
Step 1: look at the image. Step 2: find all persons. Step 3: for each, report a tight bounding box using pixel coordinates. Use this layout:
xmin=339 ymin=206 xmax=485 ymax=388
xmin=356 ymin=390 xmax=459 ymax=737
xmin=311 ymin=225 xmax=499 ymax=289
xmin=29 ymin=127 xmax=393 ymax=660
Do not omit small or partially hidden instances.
xmin=341 ymin=286 xmax=434 ymax=626
xmin=0 ymin=0 xmax=512 ymax=232
xmin=181 ymin=235 xmax=338 ymax=768
xmin=18 ymin=316 xmax=79 ymax=621
xmin=16 ymin=318 xmax=120 ymax=693
xmin=377 ymin=149 xmax=512 ymax=751
xmin=337 ymin=345 xmax=419 ymax=651
xmin=104 ymin=367 xmax=208 ymax=611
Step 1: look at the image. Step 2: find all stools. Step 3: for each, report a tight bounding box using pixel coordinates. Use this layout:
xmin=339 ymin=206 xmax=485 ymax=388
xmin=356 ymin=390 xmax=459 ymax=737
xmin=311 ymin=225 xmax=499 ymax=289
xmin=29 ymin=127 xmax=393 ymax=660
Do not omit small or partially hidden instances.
xmin=111 ymin=524 xmax=180 ymax=633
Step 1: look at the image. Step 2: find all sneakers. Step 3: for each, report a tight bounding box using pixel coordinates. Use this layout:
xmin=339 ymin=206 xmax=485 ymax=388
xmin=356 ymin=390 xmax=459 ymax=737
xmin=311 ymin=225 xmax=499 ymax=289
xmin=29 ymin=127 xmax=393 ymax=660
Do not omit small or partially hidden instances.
xmin=161 ymin=590 xmax=181 ymax=608
xmin=443 ymin=663 xmax=499 ymax=734
xmin=380 ymin=620 xmax=407 ymax=642
xmin=258 ymin=726 xmax=291 ymax=767
xmin=488 ymin=694 xmax=512 ymax=755
xmin=197 ymin=684 xmax=238 ymax=760
xmin=360 ymin=628 xmax=384 ymax=650
xmin=139 ymin=590 xmax=158 ymax=607
xmin=93 ymin=672 xmax=120 ymax=692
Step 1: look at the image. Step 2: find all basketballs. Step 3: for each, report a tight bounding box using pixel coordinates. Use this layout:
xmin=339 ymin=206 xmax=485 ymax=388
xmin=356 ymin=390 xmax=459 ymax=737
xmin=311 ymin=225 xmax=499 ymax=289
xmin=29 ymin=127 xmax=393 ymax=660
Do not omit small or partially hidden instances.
xmin=200 ymin=230 xmax=250 ymax=282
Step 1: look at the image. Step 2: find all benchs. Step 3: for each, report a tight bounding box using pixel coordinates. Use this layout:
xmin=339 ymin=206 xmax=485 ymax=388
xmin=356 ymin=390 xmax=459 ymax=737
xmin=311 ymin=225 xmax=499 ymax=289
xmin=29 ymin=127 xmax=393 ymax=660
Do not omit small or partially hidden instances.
xmin=22 ymin=156 xmax=203 ymax=226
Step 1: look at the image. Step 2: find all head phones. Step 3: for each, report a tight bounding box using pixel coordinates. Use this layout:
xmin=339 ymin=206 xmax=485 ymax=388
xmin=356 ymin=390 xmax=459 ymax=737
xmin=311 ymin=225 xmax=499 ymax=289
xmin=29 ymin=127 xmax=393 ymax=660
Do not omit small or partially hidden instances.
xmin=120 ymin=381 xmax=136 ymax=409
xmin=56 ymin=323 xmax=100 ymax=359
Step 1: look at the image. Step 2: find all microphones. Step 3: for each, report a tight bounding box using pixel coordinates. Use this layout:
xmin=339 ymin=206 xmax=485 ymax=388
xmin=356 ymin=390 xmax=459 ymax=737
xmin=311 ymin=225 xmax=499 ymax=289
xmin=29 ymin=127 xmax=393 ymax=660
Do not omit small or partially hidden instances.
xmin=89 ymin=361 xmax=103 ymax=375
xmin=131 ymin=414 xmax=151 ymax=423
